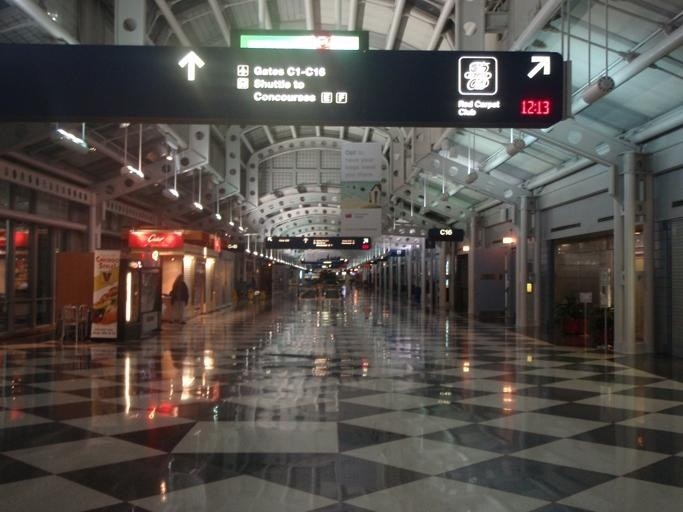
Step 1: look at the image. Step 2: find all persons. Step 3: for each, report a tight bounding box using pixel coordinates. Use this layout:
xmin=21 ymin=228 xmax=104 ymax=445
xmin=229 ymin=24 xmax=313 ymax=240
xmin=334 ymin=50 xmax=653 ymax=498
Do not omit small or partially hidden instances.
xmin=170 ymin=274 xmax=189 ymax=325
xmin=343 ymin=270 xmax=362 ymax=296
